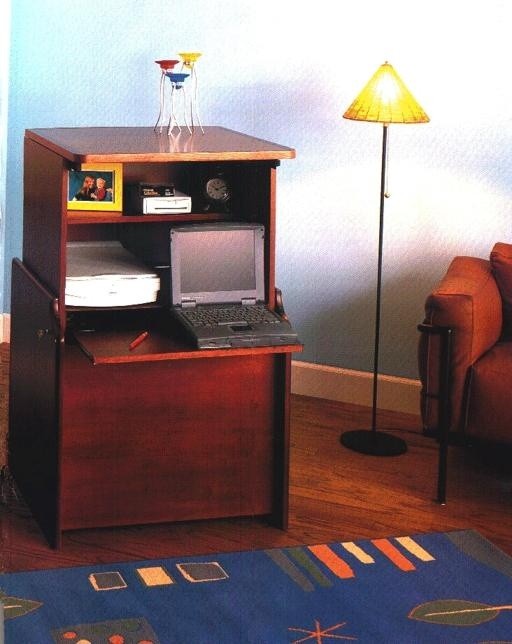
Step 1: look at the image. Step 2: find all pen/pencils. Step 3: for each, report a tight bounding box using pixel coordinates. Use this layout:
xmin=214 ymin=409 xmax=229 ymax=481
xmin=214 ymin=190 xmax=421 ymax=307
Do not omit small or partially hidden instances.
xmin=127 ymin=328 xmax=151 ymax=351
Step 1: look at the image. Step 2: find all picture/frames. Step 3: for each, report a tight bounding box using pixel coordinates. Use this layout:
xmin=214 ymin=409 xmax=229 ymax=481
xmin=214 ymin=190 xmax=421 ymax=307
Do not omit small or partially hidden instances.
xmin=67 ymin=162 xmax=124 ymax=213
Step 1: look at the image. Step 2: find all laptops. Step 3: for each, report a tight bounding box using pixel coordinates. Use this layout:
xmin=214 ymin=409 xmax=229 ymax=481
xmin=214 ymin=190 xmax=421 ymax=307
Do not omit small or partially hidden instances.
xmin=170 ymin=220 xmax=300 ymax=352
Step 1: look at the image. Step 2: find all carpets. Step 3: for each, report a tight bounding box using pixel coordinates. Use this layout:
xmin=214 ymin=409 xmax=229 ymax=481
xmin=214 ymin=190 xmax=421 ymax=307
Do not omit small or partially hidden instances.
xmin=0 ymin=528 xmax=511 ymax=644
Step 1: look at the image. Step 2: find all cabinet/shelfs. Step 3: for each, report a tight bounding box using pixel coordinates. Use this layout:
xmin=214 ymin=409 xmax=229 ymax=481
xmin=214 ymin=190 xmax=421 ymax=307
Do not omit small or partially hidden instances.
xmin=8 ymin=126 xmax=306 ymax=553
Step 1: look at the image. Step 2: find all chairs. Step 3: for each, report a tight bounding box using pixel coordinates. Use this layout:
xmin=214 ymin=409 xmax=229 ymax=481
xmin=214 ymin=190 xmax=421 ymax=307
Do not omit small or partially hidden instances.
xmin=415 ymin=242 xmax=512 ymax=507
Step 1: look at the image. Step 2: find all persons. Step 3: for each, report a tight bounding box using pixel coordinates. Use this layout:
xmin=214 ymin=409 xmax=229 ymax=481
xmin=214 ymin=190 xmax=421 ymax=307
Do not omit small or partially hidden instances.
xmin=90 ymin=177 xmax=107 ymax=201
xmin=71 ymin=175 xmax=112 ymax=202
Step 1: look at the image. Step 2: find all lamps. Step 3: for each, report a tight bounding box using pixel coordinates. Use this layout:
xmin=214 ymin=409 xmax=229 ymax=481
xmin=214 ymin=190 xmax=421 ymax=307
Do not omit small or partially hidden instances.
xmin=340 ymin=59 xmax=429 ymax=457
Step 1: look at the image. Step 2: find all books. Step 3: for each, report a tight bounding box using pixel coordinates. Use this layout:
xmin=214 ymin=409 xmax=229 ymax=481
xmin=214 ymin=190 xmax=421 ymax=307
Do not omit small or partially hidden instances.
xmin=64 ymin=239 xmax=160 ymax=308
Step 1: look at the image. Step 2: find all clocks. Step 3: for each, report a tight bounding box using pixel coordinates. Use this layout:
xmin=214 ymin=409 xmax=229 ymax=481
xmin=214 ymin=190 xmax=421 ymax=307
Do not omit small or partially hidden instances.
xmin=199 ymin=164 xmax=232 ymax=213
